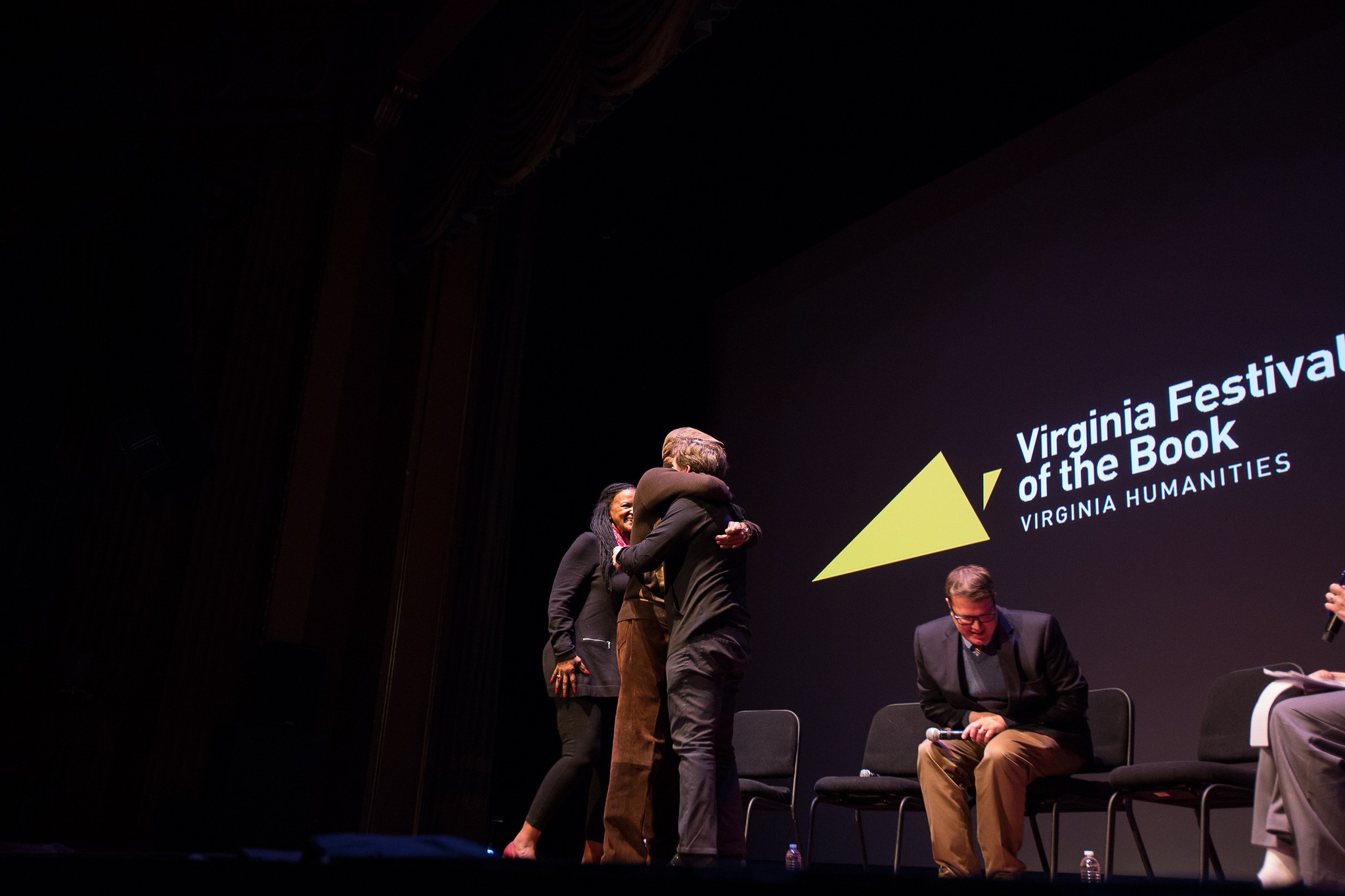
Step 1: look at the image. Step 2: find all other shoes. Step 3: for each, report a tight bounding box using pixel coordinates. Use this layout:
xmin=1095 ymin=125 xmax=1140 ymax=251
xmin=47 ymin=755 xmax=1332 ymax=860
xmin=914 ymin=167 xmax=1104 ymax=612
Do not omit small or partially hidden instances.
xmin=502 ymin=841 xmax=519 ymax=859
xmin=667 ymin=853 xmax=717 ymax=866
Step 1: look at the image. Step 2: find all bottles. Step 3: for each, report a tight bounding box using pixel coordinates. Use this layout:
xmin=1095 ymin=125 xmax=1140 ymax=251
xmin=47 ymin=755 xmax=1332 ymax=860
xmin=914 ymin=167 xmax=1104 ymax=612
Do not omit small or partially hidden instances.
xmin=786 ymin=843 xmax=803 ymax=869
xmin=1081 ymin=850 xmax=1101 ymax=882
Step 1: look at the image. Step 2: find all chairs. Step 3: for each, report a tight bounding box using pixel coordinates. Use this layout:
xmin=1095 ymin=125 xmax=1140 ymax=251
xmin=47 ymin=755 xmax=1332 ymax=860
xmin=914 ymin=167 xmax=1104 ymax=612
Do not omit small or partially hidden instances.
xmin=965 ymin=686 xmax=1152 ymax=884
xmin=807 ymin=701 xmax=926 ymax=874
xmin=731 ymin=708 xmax=804 ymax=865
xmin=1104 ymin=662 xmax=1306 ymax=888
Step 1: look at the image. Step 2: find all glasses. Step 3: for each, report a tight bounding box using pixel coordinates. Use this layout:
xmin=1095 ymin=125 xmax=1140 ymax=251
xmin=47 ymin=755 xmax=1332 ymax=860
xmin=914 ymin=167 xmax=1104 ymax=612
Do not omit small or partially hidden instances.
xmin=950 ymin=601 xmax=996 ymax=625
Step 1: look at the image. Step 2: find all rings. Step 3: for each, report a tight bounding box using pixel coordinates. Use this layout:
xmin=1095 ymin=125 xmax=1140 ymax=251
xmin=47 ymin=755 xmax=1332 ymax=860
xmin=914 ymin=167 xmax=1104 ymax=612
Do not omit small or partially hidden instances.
xmin=557 ymin=674 xmax=562 ymax=677
xmin=978 ymin=728 xmax=987 ymax=733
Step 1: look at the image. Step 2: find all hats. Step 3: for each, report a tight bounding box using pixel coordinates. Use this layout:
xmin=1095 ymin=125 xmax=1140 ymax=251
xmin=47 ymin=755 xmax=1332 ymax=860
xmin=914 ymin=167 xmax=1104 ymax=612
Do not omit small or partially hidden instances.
xmin=662 ymin=427 xmax=724 ymax=460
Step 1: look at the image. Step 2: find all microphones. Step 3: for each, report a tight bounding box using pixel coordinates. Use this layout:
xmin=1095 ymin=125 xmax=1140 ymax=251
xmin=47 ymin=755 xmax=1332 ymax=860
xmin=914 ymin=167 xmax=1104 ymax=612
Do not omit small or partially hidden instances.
xmin=926 ymin=727 xmax=972 ymax=741
xmin=1324 ymin=571 xmax=1344 ymax=641
xmin=861 ymin=769 xmax=881 ymax=776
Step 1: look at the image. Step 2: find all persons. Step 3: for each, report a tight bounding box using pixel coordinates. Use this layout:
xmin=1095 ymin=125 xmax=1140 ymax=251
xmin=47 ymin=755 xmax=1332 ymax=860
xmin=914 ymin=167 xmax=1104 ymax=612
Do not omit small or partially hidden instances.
xmin=1244 ymin=583 xmax=1345 ymax=890
xmin=503 ymin=482 xmax=636 ymax=865
xmin=911 ymin=564 xmax=1093 ymax=880
xmin=599 ymin=427 xmax=763 ymax=865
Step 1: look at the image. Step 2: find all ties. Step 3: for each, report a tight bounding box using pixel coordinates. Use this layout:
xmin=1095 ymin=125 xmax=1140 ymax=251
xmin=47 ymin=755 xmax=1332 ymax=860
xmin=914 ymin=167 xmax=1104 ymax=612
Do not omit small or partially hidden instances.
xmin=974 ymin=644 xmax=983 ymax=655
